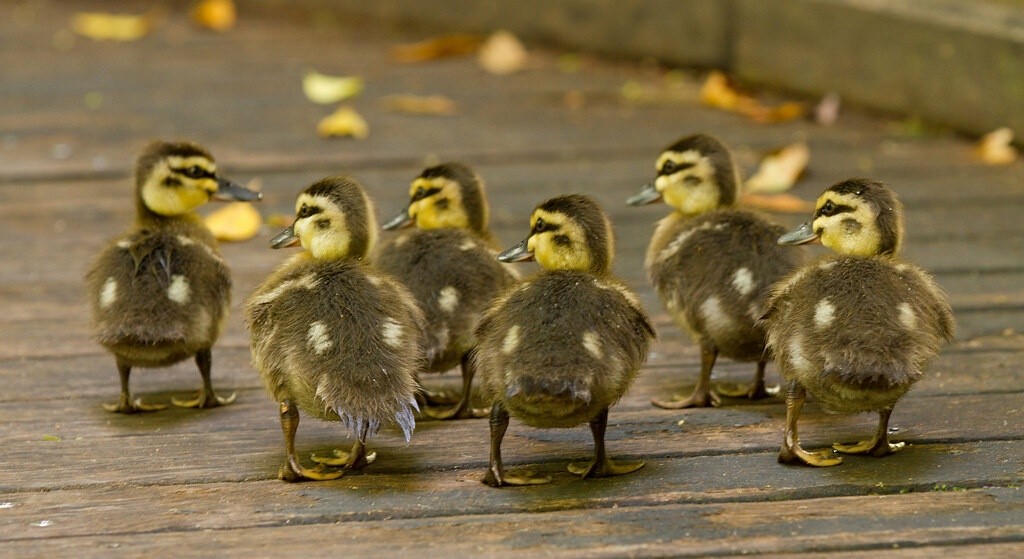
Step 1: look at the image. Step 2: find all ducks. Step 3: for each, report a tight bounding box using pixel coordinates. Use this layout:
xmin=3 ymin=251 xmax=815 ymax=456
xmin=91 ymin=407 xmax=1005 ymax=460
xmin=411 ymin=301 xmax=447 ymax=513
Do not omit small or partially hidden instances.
xmin=86 ymin=133 xmax=956 ymax=489
